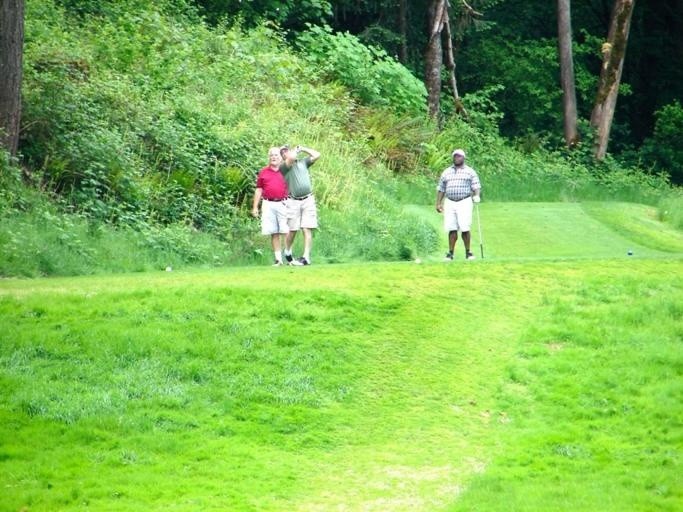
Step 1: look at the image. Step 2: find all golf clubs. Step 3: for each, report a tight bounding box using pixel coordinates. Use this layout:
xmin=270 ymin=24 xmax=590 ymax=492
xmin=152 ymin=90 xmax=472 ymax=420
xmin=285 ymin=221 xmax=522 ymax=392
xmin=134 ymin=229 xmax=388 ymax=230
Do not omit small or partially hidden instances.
xmin=476 ymin=202 xmax=485 ymax=258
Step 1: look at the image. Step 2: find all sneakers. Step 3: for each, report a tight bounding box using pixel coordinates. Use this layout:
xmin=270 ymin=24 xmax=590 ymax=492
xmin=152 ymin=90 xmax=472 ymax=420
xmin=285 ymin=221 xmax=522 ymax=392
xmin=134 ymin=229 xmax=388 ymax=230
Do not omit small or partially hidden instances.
xmin=272 ymin=250 xmax=309 ymax=267
xmin=443 ymin=252 xmax=475 ymax=261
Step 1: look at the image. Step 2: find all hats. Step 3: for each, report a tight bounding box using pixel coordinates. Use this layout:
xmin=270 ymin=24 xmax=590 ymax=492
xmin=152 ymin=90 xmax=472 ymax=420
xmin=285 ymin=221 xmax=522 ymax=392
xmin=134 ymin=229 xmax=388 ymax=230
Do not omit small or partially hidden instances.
xmin=452 ymin=149 xmax=465 ymax=157
xmin=279 ymin=146 xmax=289 ymax=154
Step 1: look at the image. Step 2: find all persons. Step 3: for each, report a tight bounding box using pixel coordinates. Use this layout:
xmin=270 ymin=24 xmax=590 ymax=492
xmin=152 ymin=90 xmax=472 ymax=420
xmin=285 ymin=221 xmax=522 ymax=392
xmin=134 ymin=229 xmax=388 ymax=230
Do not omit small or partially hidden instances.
xmin=278 ymin=145 xmax=322 ymax=266
xmin=251 ymin=146 xmax=290 ymax=267
xmin=435 ymin=148 xmax=481 ymax=261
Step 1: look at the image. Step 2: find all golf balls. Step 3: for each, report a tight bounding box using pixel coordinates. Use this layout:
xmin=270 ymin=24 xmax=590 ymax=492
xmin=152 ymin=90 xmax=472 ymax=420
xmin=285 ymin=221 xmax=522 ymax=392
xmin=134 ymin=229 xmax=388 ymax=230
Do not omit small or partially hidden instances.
xmin=416 ymin=258 xmax=421 ymax=264
xmin=165 ymin=267 xmax=171 ymax=272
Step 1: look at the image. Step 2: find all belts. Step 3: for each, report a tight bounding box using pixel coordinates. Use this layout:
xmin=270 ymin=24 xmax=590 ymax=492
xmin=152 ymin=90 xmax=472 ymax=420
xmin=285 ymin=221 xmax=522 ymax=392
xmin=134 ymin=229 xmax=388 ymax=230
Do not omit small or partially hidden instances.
xmin=263 ymin=195 xmax=308 ymax=202
xmin=446 ymin=195 xmax=472 ymax=203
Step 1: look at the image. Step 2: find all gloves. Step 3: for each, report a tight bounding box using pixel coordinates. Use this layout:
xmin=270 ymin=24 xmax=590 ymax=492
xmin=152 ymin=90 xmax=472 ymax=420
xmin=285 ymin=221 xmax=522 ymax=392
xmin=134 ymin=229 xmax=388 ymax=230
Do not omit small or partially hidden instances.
xmin=472 ymin=195 xmax=481 ymax=203
